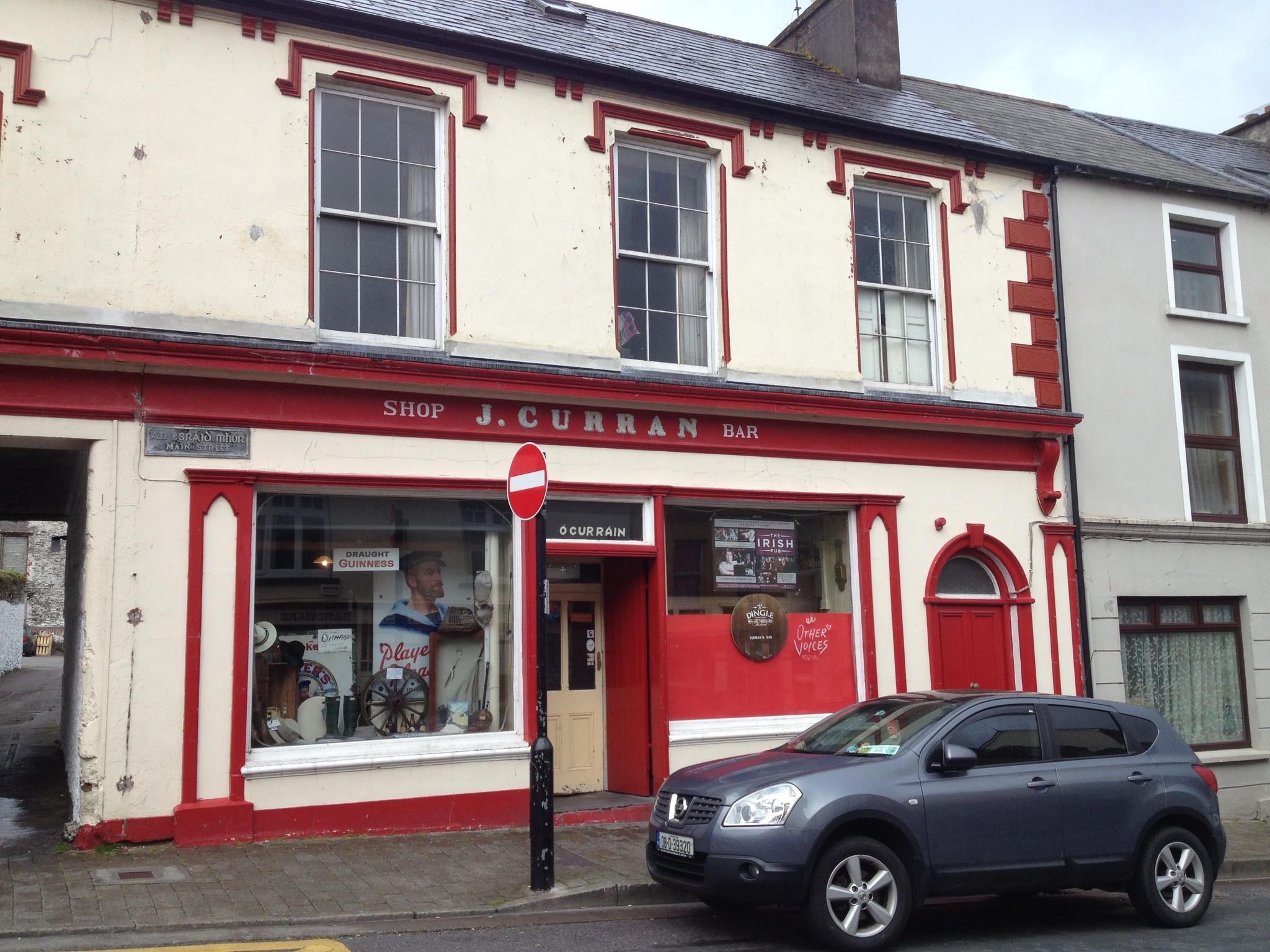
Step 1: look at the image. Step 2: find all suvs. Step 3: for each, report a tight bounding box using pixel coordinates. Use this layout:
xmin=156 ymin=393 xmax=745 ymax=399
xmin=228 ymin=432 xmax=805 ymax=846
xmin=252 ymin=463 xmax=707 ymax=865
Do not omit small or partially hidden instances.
xmin=645 ymin=689 xmax=1226 ymax=951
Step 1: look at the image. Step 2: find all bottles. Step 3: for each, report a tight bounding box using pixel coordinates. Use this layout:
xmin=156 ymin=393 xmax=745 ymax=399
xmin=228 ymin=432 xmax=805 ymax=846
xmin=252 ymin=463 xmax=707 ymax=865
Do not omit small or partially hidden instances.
xmin=385 ymin=714 xmax=394 ymax=736
xmin=418 ymin=713 xmax=426 ymax=731
xmin=409 ymin=713 xmax=416 ymax=732
xmin=400 ymin=714 xmax=407 ymax=733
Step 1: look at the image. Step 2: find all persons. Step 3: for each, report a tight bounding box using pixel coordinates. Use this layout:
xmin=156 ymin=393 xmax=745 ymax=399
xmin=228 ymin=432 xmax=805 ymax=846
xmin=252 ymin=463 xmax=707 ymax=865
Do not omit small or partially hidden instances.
xmin=715 ymin=526 xmax=796 ymax=583
xmin=378 ymin=549 xmax=448 ymax=634
xmin=296 ymin=679 xmax=310 ymax=705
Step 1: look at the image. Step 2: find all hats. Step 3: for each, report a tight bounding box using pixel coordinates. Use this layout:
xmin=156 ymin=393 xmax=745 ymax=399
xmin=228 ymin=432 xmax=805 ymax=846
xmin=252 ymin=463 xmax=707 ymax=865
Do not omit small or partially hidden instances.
xmin=398 ymin=550 xmax=447 ymax=571
xmin=253 ymin=621 xmax=277 ymax=653
xmin=298 ymin=680 xmax=311 ymax=688
xmin=280 ymin=640 xmax=306 ymax=668
xmin=268 ymin=716 xmax=301 ymax=746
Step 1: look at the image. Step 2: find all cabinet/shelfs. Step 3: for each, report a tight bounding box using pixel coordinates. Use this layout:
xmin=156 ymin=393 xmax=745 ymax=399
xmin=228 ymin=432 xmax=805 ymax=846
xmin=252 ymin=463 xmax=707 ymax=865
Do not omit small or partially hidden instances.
xmin=255 ymin=660 xmax=299 ymax=746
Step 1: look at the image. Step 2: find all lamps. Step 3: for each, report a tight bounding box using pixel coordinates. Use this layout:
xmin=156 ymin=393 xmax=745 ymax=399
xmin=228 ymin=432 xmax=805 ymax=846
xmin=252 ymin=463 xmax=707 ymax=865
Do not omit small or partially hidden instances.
xmin=313 ymin=555 xmax=333 ymax=565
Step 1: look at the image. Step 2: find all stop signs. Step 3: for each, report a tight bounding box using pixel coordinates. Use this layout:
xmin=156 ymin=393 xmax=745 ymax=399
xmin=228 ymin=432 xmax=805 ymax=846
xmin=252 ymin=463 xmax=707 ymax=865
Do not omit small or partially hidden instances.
xmin=506 ymin=442 xmax=548 ymax=520
